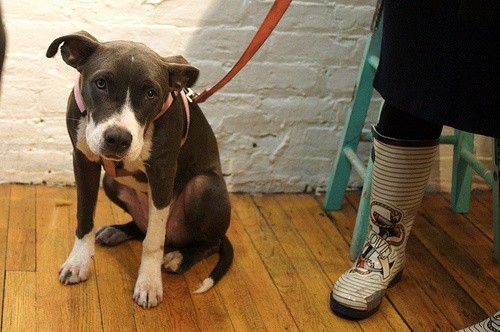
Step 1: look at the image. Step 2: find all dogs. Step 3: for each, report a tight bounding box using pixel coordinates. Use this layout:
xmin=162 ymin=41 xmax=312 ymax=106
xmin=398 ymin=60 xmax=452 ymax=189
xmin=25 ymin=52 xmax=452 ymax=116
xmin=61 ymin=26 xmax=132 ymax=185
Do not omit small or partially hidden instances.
xmin=46 ymin=30 xmax=234 ymax=310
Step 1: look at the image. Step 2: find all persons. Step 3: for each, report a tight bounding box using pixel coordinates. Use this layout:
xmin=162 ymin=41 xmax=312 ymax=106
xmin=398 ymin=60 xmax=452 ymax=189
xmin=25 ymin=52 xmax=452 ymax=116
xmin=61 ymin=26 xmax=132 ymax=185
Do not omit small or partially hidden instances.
xmin=327 ymin=0 xmax=500 ymax=332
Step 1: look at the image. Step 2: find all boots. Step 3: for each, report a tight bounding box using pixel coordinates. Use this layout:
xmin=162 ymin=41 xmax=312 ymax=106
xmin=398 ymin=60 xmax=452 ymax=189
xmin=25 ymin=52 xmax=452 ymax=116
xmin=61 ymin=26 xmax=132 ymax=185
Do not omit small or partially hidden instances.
xmin=455 ymin=310 xmax=499 ymax=332
xmin=329 ymin=121 xmax=441 ymax=322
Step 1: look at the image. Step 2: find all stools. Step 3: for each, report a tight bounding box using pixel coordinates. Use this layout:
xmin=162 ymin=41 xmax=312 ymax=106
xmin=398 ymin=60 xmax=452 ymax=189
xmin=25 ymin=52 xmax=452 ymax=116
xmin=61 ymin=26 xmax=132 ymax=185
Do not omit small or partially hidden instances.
xmin=324 ymin=0 xmax=500 ymax=262
